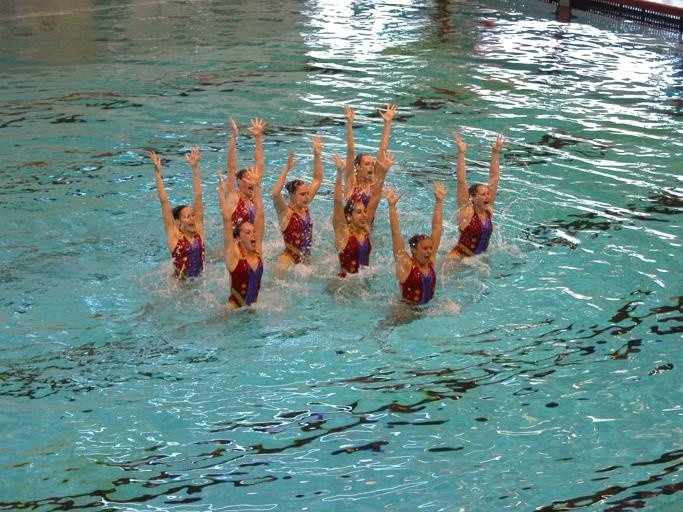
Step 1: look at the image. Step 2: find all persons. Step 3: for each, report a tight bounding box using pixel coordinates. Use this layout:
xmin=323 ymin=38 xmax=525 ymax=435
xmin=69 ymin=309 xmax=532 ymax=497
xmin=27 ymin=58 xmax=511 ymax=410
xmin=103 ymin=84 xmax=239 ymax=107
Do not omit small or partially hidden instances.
xmin=146 ymin=144 xmax=203 ymax=282
xmin=341 ymin=101 xmax=398 ymax=210
xmin=371 ymin=180 xmax=450 ymax=355
xmin=436 ymin=129 xmax=505 ymax=281
xmin=214 ymin=168 xmax=264 ymax=314
xmin=273 ymin=137 xmax=322 ymax=284
xmin=328 ymin=150 xmax=394 ymax=302
xmin=224 ymin=116 xmax=268 ymax=241
